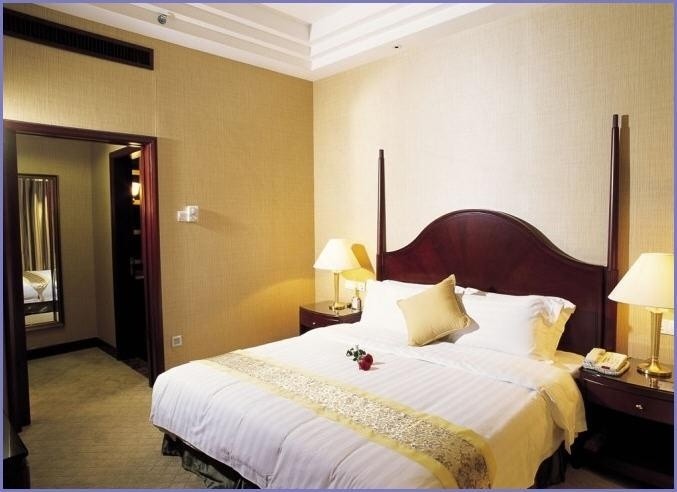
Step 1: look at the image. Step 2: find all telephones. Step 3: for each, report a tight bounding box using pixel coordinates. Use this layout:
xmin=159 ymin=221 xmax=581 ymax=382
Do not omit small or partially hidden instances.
xmin=582 ymin=348 xmax=630 ymax=376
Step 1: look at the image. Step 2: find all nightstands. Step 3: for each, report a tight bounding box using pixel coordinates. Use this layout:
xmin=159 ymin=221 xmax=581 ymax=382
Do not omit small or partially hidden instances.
xmin=299 ymin=300 xmax=362 ymax=338
xmin=558 ymin=350 xmax=675 ymax=491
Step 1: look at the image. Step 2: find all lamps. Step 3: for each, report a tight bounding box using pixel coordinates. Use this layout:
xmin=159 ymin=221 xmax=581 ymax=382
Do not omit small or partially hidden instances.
xmin=606 ymin=253 xmax=675 ymax=378
xmin=313 ymin=238 xmax=362 ymax=310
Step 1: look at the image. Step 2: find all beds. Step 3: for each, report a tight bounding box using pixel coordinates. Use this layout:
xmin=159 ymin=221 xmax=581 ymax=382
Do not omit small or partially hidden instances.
xmin=154 ymin=112 xmax=620 ymax=490
xmin=22 ymin=269 xmax=53 ymax=315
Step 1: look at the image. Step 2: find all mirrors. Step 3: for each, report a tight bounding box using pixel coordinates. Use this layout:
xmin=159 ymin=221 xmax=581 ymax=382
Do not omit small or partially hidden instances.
xmin=18 ymin=173 xmax=65 ymax=333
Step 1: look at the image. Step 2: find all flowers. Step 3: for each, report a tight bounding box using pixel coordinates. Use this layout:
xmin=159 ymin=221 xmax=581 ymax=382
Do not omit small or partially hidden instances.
xmin=345 ymin=344 xmax=373 ymax=369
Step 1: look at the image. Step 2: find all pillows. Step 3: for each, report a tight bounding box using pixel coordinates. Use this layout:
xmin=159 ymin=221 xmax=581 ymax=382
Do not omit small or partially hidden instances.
xmin=368 ymin=270 xmax=579 ymax=366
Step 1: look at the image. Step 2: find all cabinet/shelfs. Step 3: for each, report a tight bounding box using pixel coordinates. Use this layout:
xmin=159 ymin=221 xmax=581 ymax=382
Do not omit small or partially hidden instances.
xmin=2 ymin=412 xmax=30 ymax=490
